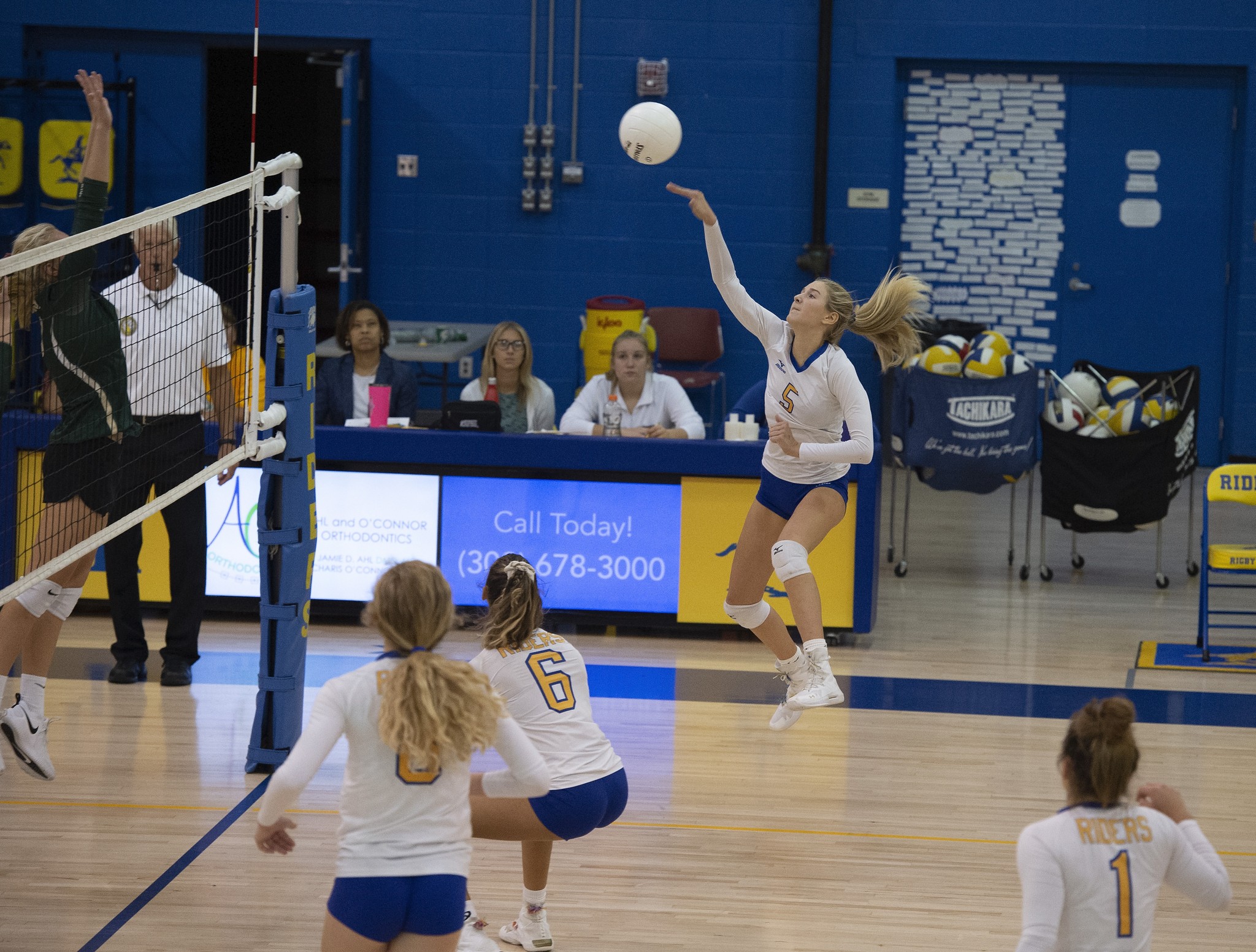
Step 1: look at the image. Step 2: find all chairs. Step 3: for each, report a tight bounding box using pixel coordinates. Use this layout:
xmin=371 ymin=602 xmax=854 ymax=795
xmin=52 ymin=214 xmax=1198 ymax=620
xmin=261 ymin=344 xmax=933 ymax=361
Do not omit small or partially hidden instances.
xmin=1196 ymin=463 xmax=1256 ymax=662
xmin=651 ymin=308 xmax=728 ymax=439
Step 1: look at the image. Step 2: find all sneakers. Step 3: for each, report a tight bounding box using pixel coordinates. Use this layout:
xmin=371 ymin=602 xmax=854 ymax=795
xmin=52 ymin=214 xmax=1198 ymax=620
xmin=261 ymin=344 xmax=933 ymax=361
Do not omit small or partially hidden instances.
xmin=498 ymin=896 xmax=554 ymax=952
xmin=786 ymin=646 xmax=845 ymax=711
xmin=0 ymin=692 xmax=61 ymax=781
xmin=455 ymin=915 xmax=501 ymax=952
xmin=768 ymin=651 xmax=817 ymax=731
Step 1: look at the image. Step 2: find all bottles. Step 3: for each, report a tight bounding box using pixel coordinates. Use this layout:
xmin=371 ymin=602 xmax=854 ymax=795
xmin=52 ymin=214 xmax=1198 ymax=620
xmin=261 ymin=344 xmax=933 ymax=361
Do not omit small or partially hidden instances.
xmin=724 ymin=413 xmax=760 ymax=441
xmin=484 ymin=377 xmax=498 ymax=404
xmin=602 ymin=395 xmax=622 ymax=437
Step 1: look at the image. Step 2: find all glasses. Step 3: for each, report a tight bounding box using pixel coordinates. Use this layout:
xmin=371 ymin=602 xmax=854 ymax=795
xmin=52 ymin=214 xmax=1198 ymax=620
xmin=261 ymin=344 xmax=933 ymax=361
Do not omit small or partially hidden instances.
xmin=495 ymin=339 xmax=525 ymax=352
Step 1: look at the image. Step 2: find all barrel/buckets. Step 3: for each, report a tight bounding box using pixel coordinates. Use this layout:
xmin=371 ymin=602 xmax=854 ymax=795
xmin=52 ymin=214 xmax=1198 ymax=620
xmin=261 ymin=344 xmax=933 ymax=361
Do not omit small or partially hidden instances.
xmin=578 ymin=295 xmax=656 ymax=385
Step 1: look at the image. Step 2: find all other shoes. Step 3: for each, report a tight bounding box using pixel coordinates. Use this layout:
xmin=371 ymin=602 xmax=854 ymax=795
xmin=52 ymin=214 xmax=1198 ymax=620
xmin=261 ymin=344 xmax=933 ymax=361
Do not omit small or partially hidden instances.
xmin=108 ymin=658 xmax=148 ymax=684
xmin=160 ymin=658 xmax=192 ymax=686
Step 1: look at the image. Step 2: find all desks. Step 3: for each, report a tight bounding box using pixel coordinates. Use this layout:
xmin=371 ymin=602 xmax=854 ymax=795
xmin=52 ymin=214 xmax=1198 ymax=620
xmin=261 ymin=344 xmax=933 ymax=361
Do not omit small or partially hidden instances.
xmin=0 ymin=414 xmax=884 ymax=646
xmin=316 ymin=322 xmax=496 ymax=420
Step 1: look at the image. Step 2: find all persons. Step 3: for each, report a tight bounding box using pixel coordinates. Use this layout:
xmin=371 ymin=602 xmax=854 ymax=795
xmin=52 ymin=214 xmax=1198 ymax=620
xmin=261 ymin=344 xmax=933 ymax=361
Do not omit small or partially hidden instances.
xmin=667 ymin=181 xmax=932 ymax=732
xmin=0 ymin=69 xmax=116 ymax=780
xmin=451 ymin=555 xmax=630 ymax=952
xmin=256 ymin=560 xmax=551 ymax=952
xmin=205 ymin=299 xmax=705 ymax=445
xmin=1014 ymin=696 xmax=1231 ymax=952
xmin=98 ymin=208 xmax=236 ymax=685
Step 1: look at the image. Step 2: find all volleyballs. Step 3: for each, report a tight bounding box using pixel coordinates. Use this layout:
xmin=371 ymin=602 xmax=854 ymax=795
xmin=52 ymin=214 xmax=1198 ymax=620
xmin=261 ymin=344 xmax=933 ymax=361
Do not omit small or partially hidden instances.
xmin=918 ymin=345 xmax=962 ymax=377
xmin=1109 ymin=398 xmax=1142 ymax=437
xmin=1088 ymin=405 xmax=1111 ymax=428
xmin=1101 ymin=376 xmax=1143 ymax=410
xmin=937 ymin=335 xmax=970 ymax=361
xmin=1077 ymin=424 xmax=1111 ymax=440
xmin=962 ymin=347 xmax=1006 ymax=380
xmin=1001 ymin=353 xmax=1032 ymax=375
xmin=1059 ymin=373 xmax=1102 ymax=414
xmin=619 ymin=101 xmax=682 ymax=165
xmin=1142 ymin=393 xmax=1180 ymax=429
xmin=902 ymin=353 xmax=923 ymax=369
xmin=970 ymin=330 xmax=1011 ymax=356
xmin=1045 ymin=398 xmax=1086 ymax=433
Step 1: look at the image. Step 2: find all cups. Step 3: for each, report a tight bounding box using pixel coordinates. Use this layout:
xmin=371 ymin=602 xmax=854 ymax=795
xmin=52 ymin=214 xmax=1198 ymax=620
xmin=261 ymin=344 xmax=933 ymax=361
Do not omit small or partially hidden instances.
xmin=368 ymin=383 xmax=392 ymax=427
